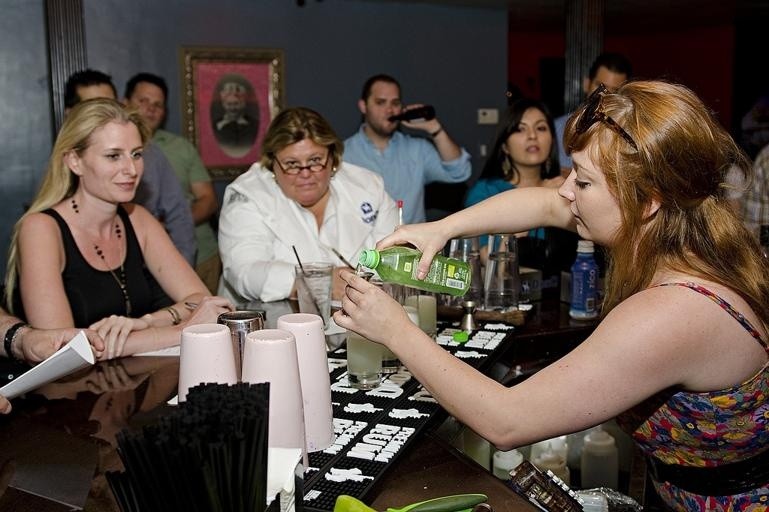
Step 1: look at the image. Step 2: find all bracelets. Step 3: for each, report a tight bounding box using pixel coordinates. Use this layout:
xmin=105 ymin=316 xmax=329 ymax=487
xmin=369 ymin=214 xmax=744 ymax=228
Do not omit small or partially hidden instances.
xmin=427 ymin=126 xmax=444 ymax=139
xmin=3 ymin=316 xmax=33 ymax=362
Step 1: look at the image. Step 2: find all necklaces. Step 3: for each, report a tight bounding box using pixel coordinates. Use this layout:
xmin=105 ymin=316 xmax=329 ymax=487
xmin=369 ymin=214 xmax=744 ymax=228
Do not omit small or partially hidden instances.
xmin=71 ymin=193 xmax=136 ymax=318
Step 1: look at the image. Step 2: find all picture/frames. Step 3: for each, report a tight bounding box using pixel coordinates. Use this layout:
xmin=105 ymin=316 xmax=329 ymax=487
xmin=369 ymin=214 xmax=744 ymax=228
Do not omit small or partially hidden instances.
xmin=177 ymin=41 xmax=291 ymax=183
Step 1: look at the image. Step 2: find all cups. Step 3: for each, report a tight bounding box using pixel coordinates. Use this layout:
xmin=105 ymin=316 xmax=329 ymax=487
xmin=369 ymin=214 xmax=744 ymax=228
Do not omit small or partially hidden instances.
xmin=340 ymin=272 xmax=374 ymax=315
xmin=244 ymin=327 xmax=309 ymax=475
xmin=276 ymin=313 xmax=335 ymax=452
xmin=487 ymin=234 xmax=522 ymax=310
xmin=395 ymin=292 xmax=419 ymax=328
xmin=217 ymin=309 xmax=266 ymax=382
xmin=177 ymin=323 xmax=239 ymax=401
xmin=345 ymin=326 xmax=383 ymax=388
xmin=405 ymin=291 xmax=437 ymax=343
xmin=296 ymin=264 xmax=332 ymax=330
xmin=446 ymin=237 xmax=483 ymax=308
xmin=381 ymin=341 xmax=399 ymax=374
xmin=462 ymin=302 xmax=478 ymax=330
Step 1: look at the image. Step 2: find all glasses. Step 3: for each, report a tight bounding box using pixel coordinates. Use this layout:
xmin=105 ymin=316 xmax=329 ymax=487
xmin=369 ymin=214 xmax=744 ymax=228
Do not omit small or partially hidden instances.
xmin=576 ymin=81 xmax=639 ymax=153
xmin=272 ymin=149 xmax=330 ymax=176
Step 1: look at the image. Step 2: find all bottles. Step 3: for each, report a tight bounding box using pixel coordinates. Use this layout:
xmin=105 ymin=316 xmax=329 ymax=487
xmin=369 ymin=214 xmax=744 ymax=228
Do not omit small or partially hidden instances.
xmin=454 ymin=425 xmax=623 ymax=491
xmin=387 ymin=103 xmax=435 ymax=124
xmin=356 ymin=247 xmax=473 ymax=296
xmin=570 ymin=239 xmax=599 ymax=322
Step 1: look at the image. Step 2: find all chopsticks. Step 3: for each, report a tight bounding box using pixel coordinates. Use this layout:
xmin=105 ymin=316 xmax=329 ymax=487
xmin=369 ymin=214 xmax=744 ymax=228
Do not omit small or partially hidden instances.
xmin=106 ymin=382 xmax=270 ymax=510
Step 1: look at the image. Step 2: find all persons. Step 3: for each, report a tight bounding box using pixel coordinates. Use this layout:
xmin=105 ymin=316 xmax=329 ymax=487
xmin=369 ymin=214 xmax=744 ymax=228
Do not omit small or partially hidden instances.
xmin=545 ymin=48 xmax=639 ymax=179
xmin=240 ymin=301 xmax=352 ymax=353
xmin=61 ymin=72 xmax=197 ymax=282
xmin=1 ymin=307 xmax=106 ymax=422
xmin=212 ymin=106 xmax=403 ymax=302
xmin=121 ymin=68 xmax=224 ymax=304
xmin=0 ymin=95 xmax=236 ymax=366
xmin=739 ymin=136 xmax=769 ymax=248
xmin=456 ymin=97 xmax=574 ymax=267
xmin=334 ymin=72 xmax=477 ymax=228
xmin=31 ymin=356 xmax=182 ymax=511
xmin=325 ymin=79 xmax=769 ymax=511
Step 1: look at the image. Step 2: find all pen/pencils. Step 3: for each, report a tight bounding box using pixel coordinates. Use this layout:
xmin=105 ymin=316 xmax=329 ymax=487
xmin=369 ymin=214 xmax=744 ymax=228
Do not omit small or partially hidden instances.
xmin=185 ymin=302 xmax=232 ymax=312
